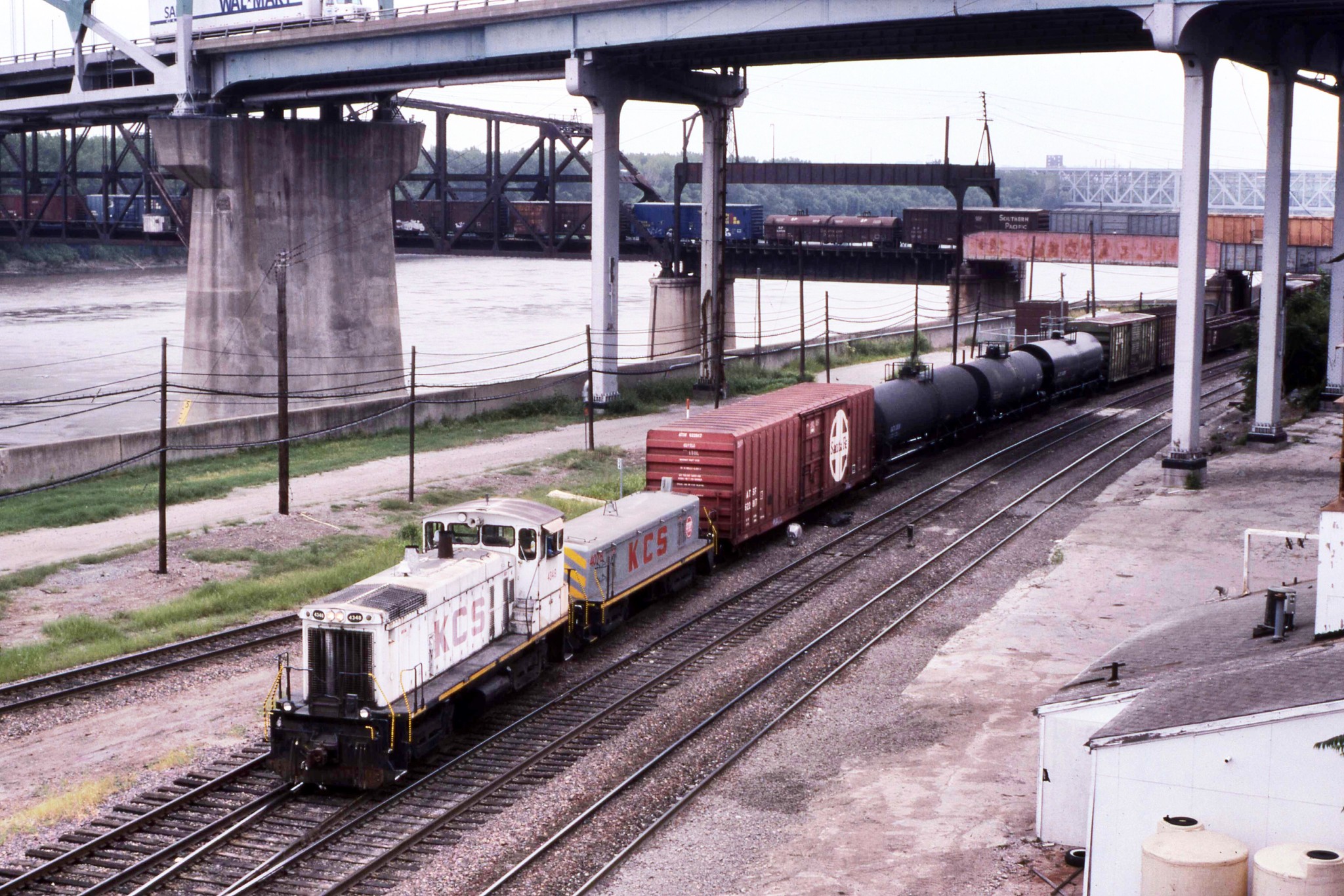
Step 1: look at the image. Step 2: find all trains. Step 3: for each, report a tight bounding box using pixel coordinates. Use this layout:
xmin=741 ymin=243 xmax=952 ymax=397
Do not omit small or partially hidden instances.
xmin=265 ymin=303 xmax=1260 ymax=795
xmin=0 ymin=189 xmax=1337 ymax=252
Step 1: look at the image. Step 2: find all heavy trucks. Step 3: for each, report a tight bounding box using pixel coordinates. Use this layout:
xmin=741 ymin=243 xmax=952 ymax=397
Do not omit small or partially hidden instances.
xmin=148 ymin=0 xmax=370 ymax=45
